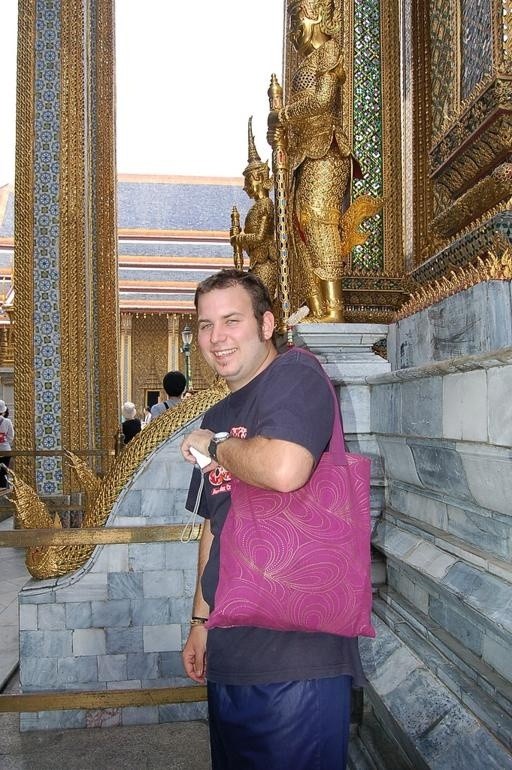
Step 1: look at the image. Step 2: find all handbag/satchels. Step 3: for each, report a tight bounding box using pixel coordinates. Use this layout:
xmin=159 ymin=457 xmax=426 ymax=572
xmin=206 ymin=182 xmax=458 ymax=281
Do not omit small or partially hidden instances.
xmin=203 ymin=452 xmax=376 ymax=638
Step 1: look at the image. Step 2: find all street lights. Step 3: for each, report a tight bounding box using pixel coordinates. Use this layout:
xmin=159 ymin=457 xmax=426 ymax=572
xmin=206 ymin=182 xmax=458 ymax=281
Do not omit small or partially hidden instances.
xmin=180 ymin=324 xmax=193 ymax=392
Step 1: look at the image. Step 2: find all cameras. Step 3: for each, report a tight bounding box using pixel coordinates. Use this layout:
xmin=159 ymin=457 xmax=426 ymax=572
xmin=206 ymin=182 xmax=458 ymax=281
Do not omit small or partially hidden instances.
xmin=187 ymin=446 xmax=210 ymax=470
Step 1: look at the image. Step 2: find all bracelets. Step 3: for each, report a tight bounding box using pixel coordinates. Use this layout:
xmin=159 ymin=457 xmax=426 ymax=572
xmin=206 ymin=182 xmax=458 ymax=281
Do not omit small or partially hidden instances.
xmin=190 ymin=619 xmax=207 ymax=625
xmin=192 ymin=616 xmax=208 ymax=622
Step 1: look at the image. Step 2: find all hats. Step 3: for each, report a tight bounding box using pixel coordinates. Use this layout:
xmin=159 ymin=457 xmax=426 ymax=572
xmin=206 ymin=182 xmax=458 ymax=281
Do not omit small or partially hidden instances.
xmin=121 ymin=401 xmax=137 ymax=419
xmin=0 ymin=400 xmax=7 ymax=413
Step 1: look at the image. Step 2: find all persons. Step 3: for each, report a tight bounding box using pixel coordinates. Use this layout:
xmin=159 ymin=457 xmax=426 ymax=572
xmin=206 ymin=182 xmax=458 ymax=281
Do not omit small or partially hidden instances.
xmin=177 ymin=269 xmax=366 ymax=769
xmin=182 ymin=389 xmax=195 ymax=399
xmin=230 ymin=159 xmax=279 ymax=302
xmin=122 ymin=402 xmax=141 ymax=444
xmin=267 ymin=0 xmax=368 ymax=325
xmin=143 ymin=405 xmax=153 ymax=426
xmin=0 ymin=399 xmax=15 ymax=489
xmin=149 ymin=370 xmax=187 ymax=421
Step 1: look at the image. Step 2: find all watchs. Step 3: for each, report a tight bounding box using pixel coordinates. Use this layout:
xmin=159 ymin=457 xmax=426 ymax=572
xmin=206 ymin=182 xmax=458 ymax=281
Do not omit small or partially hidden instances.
xmin=208 ymin=431 xmax=233 ymax=465
